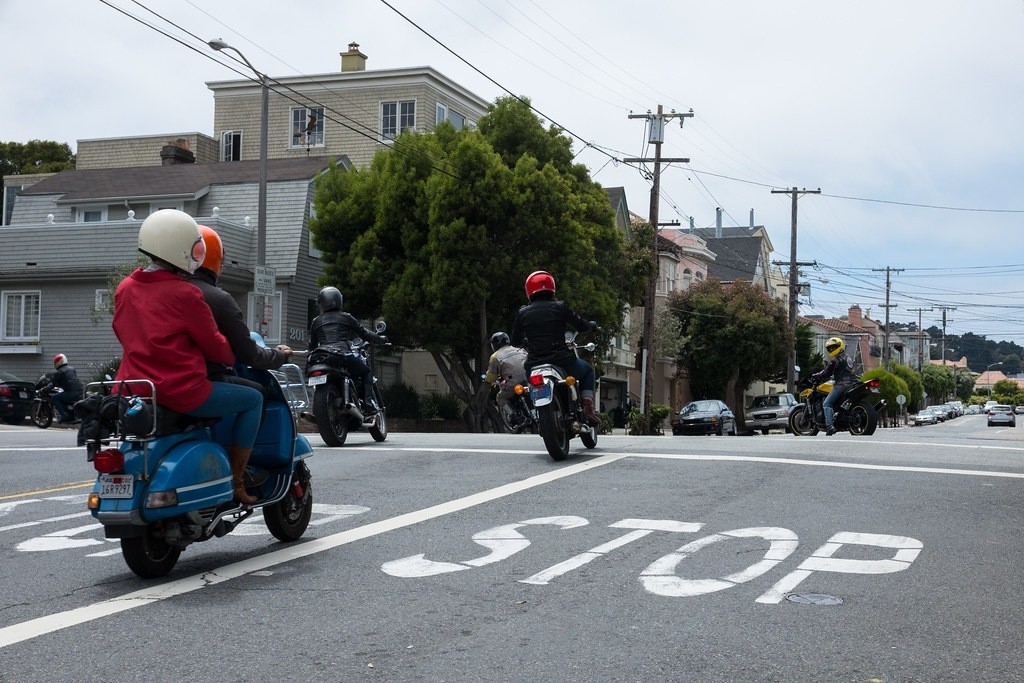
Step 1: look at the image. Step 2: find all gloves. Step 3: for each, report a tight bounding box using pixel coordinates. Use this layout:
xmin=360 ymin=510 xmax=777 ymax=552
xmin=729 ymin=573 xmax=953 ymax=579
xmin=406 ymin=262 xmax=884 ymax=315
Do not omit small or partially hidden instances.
xmin=378 ymin=335 xmax=389 ymax=345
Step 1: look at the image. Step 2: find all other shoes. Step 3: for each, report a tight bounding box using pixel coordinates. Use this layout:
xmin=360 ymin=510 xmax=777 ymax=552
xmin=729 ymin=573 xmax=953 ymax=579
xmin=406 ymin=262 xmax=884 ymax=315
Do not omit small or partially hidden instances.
xmin=509 ymin=414 xmax=525 ymax=427
xmin=58 ymin=413 xmax=75 ymax=424
xmin=244 ymin=465 xmax=269 ymax=486
xmin=826 ymin=427 xmax=836 ymax=436
xmin=363 ymin=398 xmax=377 ymax=412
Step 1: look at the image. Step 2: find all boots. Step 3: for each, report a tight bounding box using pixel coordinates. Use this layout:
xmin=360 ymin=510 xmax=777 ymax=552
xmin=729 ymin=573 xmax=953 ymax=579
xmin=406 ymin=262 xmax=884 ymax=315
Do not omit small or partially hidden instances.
xmin=582 ymin=399 xmax=602 ymax=426
xmin=231 ymin=445 xmax=257 ymax=504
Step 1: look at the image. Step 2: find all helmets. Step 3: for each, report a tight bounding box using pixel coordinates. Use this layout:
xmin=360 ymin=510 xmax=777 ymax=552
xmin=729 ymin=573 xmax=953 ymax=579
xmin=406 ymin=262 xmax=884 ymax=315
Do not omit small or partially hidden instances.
xmin=490 ymin=332 xmax=510 ymax=353
xmin=198 ymin=225 xmax=224 ymax=277
xmin=525 ymin=271 xmax=555 ymax=303
xmin=138 ymin=209 xmax=205 ymax=274
xmin=824 ymin=337 xmax=846 ymax=357
xmin=54 ymin=353 xmax=68 ymax=369
xmin=317 ymin=286 xmax=342 ymax=309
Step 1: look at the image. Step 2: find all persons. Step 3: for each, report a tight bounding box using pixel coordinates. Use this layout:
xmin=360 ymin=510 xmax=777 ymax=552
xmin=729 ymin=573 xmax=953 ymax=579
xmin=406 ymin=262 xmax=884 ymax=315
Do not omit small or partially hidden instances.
xmin=483 ymin=332 xmax=528 ymax=418
xmin=307 ymin=286 xmax=387 ymax=411
xmin=107 ymin=209 xmax=291 ymax=502
xmin=812 ymin=337 xmax=857 ymax=436
xmin=621 ymin=392 xmax=633 ymax=428
xmin=40 ymin=354 xmax=84 ymax=424
xmin=510 ymin=271 xmax=597 ymax=415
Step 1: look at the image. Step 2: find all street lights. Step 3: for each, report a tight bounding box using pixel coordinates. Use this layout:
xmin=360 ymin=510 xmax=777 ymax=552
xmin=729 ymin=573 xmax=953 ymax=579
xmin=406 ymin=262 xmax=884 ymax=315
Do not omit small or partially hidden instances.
xmin=987 ymin=362 xmax=1003 ymax=399
xmin=885 ymin=327 xmax=955 ymax=372
xmin=209 ymin=37 xmax=269 ymax=342
xmin=786 ymin=278 xmax=828 ymax=394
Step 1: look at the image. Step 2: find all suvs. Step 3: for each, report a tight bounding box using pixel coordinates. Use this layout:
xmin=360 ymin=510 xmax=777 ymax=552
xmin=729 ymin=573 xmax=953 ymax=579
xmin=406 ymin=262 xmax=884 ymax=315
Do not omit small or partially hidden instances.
xmin=744 ymin=393 xmax=798 ymax=436
xmin=985 ymin=401 xmax=998 ymax=413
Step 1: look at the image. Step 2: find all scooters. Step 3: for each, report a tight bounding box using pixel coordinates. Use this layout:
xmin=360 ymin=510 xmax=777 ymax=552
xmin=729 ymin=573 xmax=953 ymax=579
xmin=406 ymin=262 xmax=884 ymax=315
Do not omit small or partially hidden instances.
xmin=80 ymin=349 xmax=315 ymax=580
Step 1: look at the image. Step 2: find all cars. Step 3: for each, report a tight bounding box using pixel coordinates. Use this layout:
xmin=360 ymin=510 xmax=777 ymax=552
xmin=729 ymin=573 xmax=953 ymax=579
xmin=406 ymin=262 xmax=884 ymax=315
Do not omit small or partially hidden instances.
xmin=672 ymin=399 xmax=737 ymax=437
xmin=915 ymin=409 xmax=936 ymax=425
xmin=926 ymin=406 xmax=944 ymax=422
xmin=987 ymin=405 xmax=1015 ymax=427
xmin=1015 ymin=404 xmax=1024 ymax=415
xmin=969 ymin=404 xmax=981 ymax=414
xmin=940 ymin=401 xmax=973 ymax=419
xmin=0 ymin=369 xmax=36 ymax=425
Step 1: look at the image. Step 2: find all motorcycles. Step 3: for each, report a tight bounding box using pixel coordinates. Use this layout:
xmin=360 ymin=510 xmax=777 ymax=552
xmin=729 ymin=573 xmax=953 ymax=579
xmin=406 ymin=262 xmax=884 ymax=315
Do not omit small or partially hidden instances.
xmin=481 ymin=374 xmax=540 ymax=433
xmin=307 ymin=321 xmax=388 ymax=446
xmin=788 ymin=365 xmax=887 ymax=436
xmin=29 ymin=374 xmax=92 ymax=428
xmin=528 ymin=327 xmax=600 ymax=461
